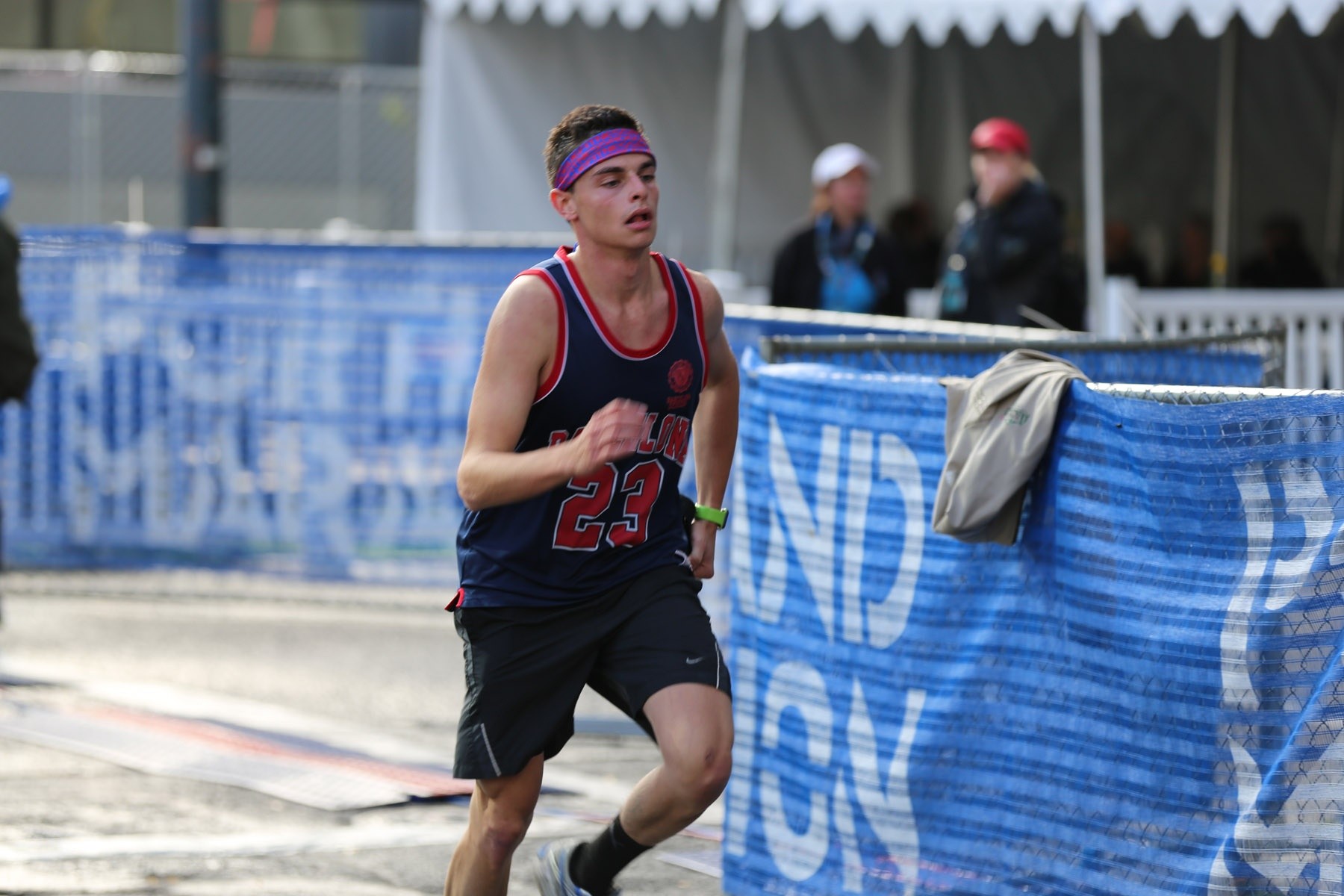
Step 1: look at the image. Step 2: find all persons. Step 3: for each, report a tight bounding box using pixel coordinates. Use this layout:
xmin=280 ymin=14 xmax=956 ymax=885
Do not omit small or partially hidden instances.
xmin=951 ymin=118 xmax=1085 ymax=333
xmin=878 ymin=171 xmax=1325 ymax=294
xmin=444 ymin=105 xmax=740 ymax=894
xmin=769 ymin=142 xmax=949 ymax=315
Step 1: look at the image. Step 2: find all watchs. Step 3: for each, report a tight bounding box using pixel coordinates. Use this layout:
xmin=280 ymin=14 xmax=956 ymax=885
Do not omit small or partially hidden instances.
xmin=693 ymin=501 xmax=730 ymax=529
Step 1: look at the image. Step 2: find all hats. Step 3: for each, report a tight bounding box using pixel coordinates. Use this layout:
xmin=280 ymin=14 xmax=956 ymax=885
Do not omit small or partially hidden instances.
xmin=811 ymin=140 xmax=877 ymax=188
xmin=969 ymin=116 xmax=1029 ymax=153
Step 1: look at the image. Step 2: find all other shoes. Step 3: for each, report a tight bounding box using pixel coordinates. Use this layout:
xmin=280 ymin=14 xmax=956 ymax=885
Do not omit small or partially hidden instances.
xmin=531 ymin=835 xmax=624 ymax=896
